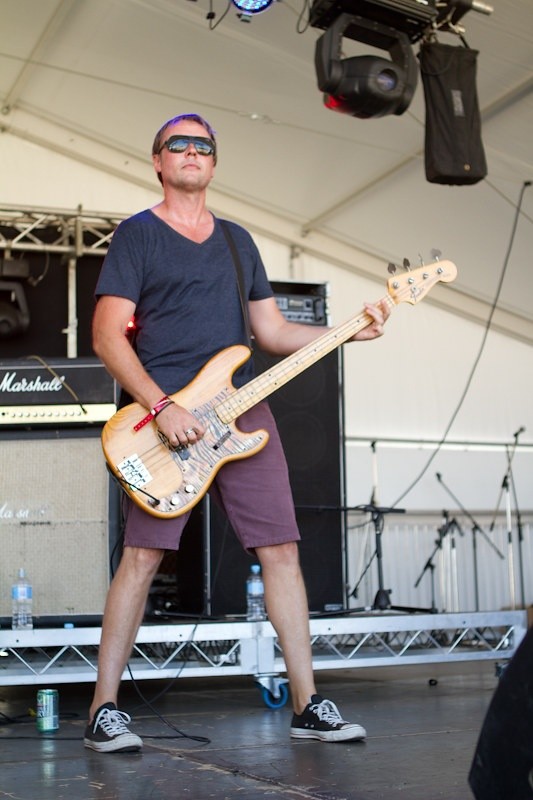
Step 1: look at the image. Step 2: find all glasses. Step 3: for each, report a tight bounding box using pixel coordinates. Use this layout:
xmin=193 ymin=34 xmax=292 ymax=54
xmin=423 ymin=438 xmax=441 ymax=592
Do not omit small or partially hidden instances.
xmin=158 ymin=135 xmax=215 ymax=156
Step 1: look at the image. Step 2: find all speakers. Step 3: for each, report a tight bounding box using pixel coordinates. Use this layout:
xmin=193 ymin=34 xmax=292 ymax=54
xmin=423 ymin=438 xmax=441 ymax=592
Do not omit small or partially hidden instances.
xmin=0 ymin=430 xmax=121 ymax=623
xmin=150 ymin=328 xmax=347 ymax=624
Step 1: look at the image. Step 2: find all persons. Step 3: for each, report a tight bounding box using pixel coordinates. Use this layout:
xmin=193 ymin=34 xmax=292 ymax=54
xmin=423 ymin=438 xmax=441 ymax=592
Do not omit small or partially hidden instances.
xmin=81 ymin=108 xmax=390 ymax=754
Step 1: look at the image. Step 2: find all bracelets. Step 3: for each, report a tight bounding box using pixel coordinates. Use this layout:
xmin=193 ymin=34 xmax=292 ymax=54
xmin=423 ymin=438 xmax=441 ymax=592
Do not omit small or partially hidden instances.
xmin=133 ymin=396 xmax=175 ymax=431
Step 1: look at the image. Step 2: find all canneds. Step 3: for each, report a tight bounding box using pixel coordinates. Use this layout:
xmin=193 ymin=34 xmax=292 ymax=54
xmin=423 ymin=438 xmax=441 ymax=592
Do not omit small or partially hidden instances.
xmin=35 ymin=688 xmax=61 ymax=736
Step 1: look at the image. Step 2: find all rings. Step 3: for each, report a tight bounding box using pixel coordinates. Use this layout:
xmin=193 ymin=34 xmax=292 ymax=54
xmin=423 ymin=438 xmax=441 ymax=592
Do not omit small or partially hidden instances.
xmin=184 ymin=428 xmax=194 ymax=436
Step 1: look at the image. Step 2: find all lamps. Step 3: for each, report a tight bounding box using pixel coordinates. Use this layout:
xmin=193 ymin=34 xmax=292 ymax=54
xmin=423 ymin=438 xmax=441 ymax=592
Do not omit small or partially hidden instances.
xmin=312 ymin=11 xmax=421 ymax=120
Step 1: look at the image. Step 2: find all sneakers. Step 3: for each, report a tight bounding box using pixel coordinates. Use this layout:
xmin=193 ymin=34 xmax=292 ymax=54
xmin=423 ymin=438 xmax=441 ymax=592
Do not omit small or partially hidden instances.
xmin=291 ymin=694 xmax=367 ymax=741
xmin=84 ymin=701 xmax=143 ymax=754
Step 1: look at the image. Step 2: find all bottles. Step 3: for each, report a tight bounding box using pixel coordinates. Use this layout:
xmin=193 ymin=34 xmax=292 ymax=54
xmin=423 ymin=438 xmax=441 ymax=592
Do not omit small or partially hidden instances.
xmin=245 ymin=563 xmax=267 ymax=622
xmin=10 ymin=567 xmax=34 ymax=631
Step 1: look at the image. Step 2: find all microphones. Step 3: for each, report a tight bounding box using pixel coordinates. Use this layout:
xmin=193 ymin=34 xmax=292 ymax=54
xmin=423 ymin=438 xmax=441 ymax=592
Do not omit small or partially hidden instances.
xmin=514 ymin=427 xmax=524 ymax=436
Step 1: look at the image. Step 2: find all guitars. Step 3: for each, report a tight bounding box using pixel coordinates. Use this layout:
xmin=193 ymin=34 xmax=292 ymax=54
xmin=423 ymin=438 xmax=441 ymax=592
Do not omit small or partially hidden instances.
xmin=102 ymin=247 xmax=456 ymax=518
